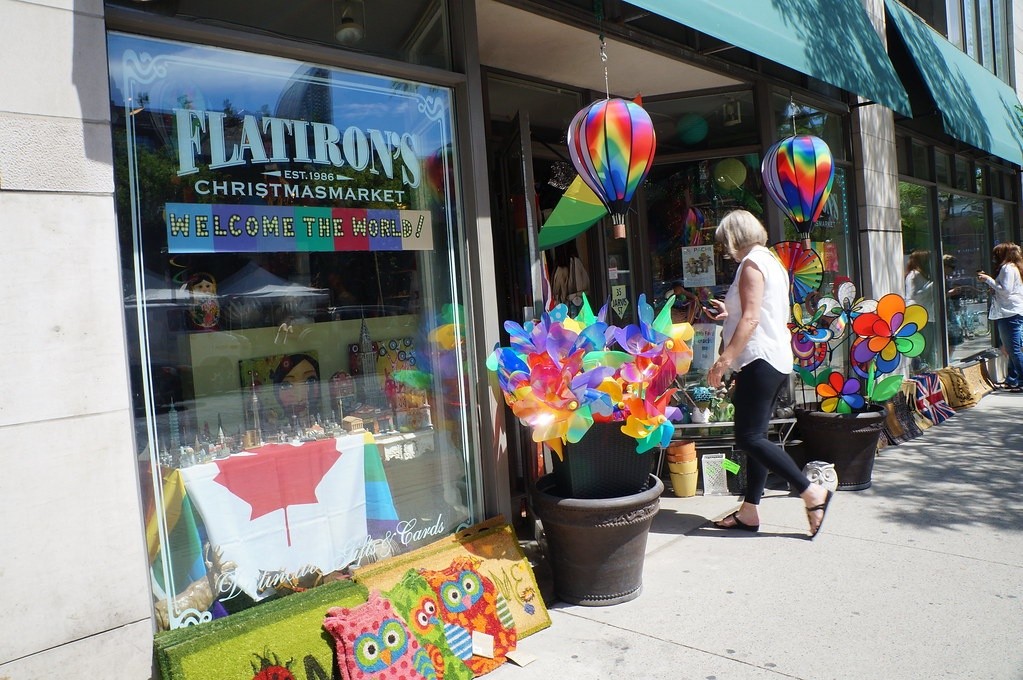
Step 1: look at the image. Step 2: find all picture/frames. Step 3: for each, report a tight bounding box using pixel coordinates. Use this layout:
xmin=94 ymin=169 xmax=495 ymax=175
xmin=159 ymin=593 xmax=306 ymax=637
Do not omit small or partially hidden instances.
xmin=347 ymin=336 xmax=418 ymax=404
xmin=239 ymin=349 xmax=324 ymax=438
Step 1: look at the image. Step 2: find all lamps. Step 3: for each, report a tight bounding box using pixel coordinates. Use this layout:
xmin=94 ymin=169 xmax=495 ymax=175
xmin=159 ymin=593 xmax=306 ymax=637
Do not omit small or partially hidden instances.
xmin=760 ymin=88 xmax=835 ymax=252
xmin=722 ymin=95 xmax=743 ymax=129
xmin=331 ymin=0 xmax=368 ymax=48
xmin=566 ymin=34 xmax=656 ymax=240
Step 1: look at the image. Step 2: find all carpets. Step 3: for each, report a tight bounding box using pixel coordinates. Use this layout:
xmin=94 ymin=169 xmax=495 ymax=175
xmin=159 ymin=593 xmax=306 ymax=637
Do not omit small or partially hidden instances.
xmin=152 ymin=512 xmax=553 ymax=680
xmin=870 ymin=362 xmax=996 ymax=457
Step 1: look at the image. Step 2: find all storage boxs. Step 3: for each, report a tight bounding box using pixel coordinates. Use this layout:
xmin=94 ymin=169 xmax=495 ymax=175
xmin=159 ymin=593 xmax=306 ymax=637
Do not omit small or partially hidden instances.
xmin=691 ymin=322 xmax=723 ymax=375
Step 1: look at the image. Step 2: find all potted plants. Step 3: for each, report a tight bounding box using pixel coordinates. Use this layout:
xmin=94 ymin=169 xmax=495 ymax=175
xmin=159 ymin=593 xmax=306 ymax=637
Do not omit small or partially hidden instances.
xmin=668 ymin=372 xmax=736 ymax=437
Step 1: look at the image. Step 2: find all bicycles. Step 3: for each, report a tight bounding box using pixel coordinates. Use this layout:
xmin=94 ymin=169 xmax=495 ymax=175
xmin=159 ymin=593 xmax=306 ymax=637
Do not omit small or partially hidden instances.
xmin=956 ymin=308 xmax=991 ymax=337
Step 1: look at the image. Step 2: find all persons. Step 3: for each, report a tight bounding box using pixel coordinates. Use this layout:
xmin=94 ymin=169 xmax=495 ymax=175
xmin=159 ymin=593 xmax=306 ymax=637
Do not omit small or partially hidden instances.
xmin=701 ymin=208 xmax=833 ymax=542
xmin=976 ymin=240 xmax=1023 ymax=393
xmin=904 ymin=250 xmax=962 ymax=354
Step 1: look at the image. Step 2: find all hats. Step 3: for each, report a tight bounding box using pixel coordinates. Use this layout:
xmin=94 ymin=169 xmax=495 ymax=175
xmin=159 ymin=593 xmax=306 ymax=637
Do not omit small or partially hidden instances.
xmin=943 ymin=254 xmax=958 ymax=268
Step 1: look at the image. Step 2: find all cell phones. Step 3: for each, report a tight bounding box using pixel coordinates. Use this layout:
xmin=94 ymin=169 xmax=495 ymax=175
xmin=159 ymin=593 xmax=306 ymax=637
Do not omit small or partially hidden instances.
xmin=698 ymin=299 xmax=719 ymax=316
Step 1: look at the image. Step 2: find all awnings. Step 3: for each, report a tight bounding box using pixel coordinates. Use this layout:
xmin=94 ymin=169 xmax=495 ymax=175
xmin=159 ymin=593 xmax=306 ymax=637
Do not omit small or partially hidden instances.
xmin=623 ymin=0 xmax=1023 ymax=169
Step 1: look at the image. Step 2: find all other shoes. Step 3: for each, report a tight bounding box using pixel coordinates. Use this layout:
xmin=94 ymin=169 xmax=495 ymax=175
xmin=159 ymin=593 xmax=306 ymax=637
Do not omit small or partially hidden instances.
xmin=1001 ymin=383 xmax=1023 ymax=392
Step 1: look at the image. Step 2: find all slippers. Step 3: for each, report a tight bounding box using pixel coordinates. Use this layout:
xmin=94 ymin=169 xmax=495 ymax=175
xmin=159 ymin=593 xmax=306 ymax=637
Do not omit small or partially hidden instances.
xmin=714 ymin=510 xmax=759 ymax=532
xmin=805 ymin=490 xmax=833 ymax=538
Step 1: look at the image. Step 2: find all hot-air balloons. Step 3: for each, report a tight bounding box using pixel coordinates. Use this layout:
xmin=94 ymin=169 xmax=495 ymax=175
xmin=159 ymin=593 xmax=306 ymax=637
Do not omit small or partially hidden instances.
xmin=760 ymin=136 xmax=839 ymax=252
xmin=565 ymin=96 xmax=658 ymax=241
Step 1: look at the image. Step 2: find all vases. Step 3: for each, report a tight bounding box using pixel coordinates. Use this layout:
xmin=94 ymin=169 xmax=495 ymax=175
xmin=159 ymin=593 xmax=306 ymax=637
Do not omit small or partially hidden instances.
xmin=532 ymin=473 xmax=665 ymax=608
xmin=666 ymin=440 xmax=698 ymax=497
xmin=793 ymin=400 xmax=888 ymax=491
